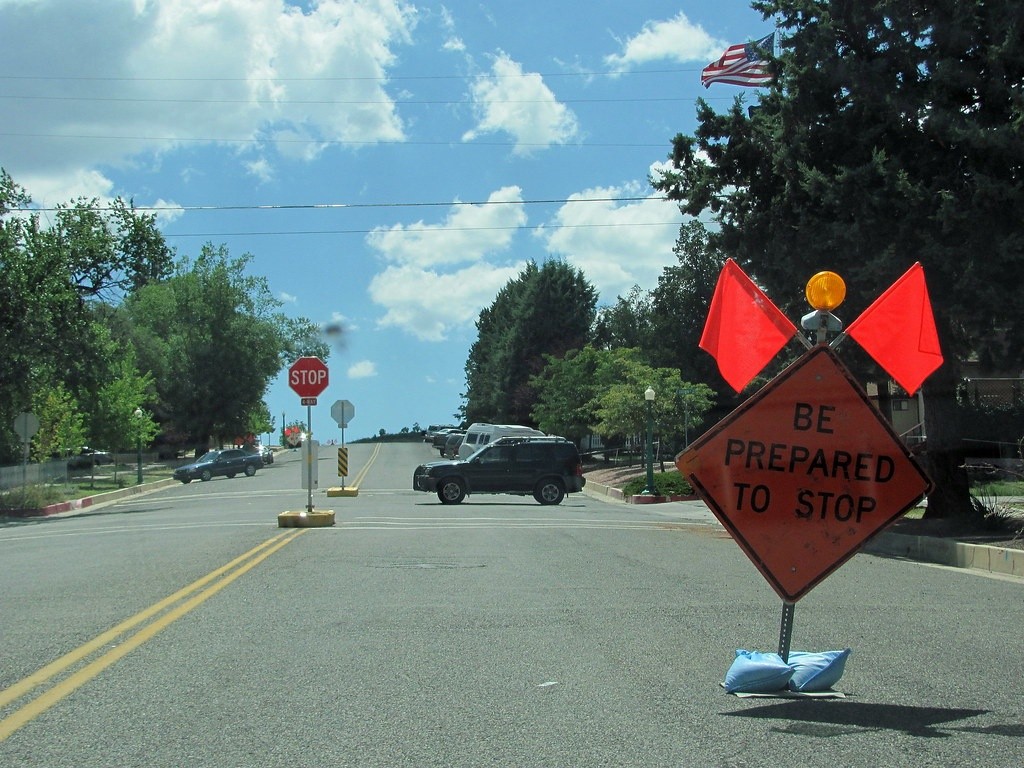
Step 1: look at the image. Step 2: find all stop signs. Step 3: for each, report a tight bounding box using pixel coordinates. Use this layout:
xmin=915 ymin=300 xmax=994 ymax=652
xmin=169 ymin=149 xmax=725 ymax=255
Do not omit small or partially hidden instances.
xmin=288 ymin=356 xmax=329 ymax=398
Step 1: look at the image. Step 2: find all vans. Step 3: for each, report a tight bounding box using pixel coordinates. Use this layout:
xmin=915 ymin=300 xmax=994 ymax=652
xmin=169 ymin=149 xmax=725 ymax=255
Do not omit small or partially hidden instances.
xmin=459 ymin=423 xmax=546 ymax=462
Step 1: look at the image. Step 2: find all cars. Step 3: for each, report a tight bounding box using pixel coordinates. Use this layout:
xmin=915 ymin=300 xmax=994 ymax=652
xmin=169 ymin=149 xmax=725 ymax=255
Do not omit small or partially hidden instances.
xmin=242 ymin=445 xmax=274 ymax=464
xmin=173 ymin=449 xmax=263 ymax=484
xmin=424 ymin=423 xmax=466 ymax=461
xmin=50 ymin=448 xmax=116 ymax=470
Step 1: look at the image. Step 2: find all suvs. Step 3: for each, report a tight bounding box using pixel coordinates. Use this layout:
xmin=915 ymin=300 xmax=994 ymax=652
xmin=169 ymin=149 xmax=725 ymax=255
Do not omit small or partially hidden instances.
xmin=412 ymin=435 xmax=586 ymax=507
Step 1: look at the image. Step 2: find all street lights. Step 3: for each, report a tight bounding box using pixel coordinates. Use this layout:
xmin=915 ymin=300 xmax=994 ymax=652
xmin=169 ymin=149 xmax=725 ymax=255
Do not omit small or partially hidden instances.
xmin=282 ymin=412 xmax=286 ymax=446
xmin=135 ymin=406 xmax=144 ymax=484
xmin=642 ymin=386 xmax=659 ymax=496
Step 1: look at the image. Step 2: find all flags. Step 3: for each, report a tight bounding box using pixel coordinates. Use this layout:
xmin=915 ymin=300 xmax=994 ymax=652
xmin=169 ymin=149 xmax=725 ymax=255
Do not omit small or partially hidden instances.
xmin=293 ymin=426 xmax=299 ymax=433
xmin=233 ymin=436 xmax=245 ymax=446
xmin=697 ymin=259 xmax=797 ymax=393
xmin=700 ymin=26 xmax=777 ymax=89
xmin=284 ymin=429 xmax=291 ymax=435
xmin=246 ymin=434 xmax=254 ymax=443
xmin=844 ymin=260 xmax=945 ymax=398
